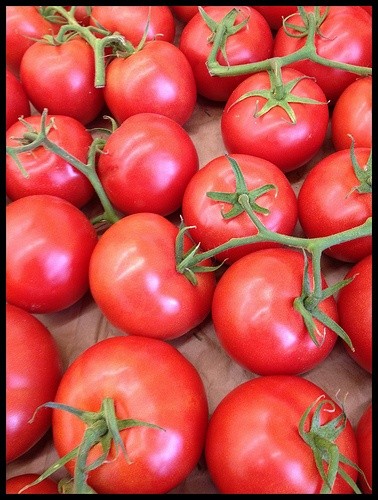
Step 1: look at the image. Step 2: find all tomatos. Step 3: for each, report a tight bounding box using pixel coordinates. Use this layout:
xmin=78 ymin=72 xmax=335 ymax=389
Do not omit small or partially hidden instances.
xmin=6 ymin=6 xmax=372 ymax=494
